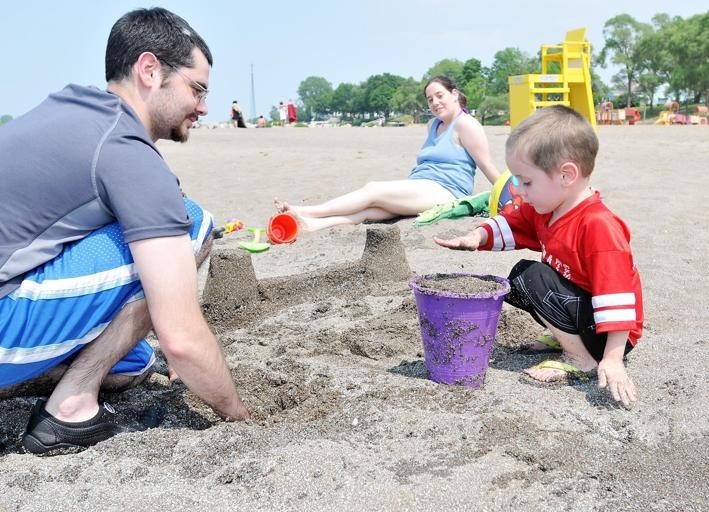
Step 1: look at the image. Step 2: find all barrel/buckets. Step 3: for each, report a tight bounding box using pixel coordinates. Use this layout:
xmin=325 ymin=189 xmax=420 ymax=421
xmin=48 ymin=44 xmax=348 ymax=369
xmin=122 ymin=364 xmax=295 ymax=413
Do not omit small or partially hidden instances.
xmin=408 ymin=271 xmax=510 ymax=386
xmin=266 ymin=213 xmax=298 ymax=245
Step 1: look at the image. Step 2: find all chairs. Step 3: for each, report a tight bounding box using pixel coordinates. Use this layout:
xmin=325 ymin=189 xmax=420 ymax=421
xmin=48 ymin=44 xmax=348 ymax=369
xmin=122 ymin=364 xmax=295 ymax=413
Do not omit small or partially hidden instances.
xmin=510 ymin=28 xmax=599 ymax=144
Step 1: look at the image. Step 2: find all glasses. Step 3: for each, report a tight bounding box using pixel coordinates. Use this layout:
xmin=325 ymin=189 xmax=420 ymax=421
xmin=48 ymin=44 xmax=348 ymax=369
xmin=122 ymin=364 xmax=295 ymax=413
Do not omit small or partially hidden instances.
xmin=162 ymin=58 xmax=209 ymax=101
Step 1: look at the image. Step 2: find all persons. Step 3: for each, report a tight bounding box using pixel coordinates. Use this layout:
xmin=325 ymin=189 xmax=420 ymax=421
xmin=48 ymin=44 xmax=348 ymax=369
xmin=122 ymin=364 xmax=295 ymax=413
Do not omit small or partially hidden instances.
xmin=433 ymin=101 xmax=644 ymax=406
xmin=600 ymin=102 xmax=613 ymax=125
xmin=231 ymin=100 xmax=248 ymax=128
xmin=273 ymin=75 xmax=501 ymax=234
xmin=279 ymin=102 xmax=288 ymax=127
xmin=630 ymin=104 xmax=640 ymax=122
xmin=1 ymin=6 xmax=250 ymax=451
xmin=279 ymin=98 xmax=298 ymax=126
xmin=255 ymin=115 xmax=266 ymax=128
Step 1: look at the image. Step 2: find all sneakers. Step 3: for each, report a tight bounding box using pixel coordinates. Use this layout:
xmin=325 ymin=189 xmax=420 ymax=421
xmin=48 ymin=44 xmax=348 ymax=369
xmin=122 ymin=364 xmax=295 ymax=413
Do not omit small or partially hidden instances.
xmin=16 ymin=399 xmax=166 ymax=457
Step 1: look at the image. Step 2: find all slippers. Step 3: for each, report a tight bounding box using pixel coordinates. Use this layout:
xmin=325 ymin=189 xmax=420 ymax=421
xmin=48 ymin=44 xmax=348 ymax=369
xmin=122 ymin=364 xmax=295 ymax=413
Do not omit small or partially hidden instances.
xmin=521 ymin=334 xmax=562 ymax=353
xmin=523 ymin=359 xmax=600 ymax=388
